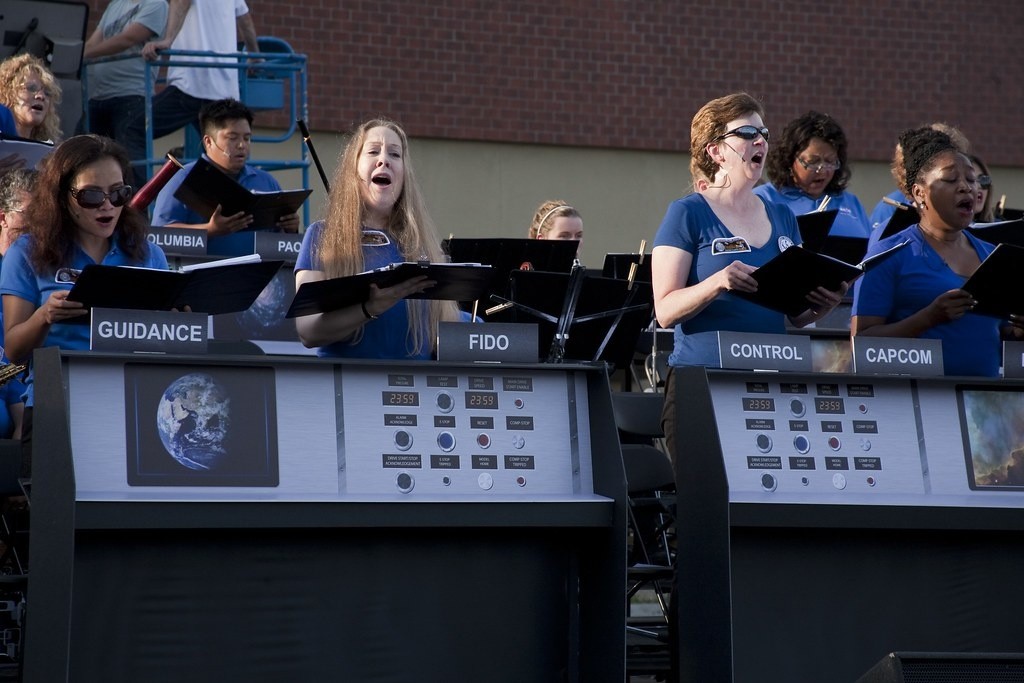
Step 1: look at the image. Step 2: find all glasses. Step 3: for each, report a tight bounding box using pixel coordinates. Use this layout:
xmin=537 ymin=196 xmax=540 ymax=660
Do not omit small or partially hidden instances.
xmin=719 ymin=125 xmax=771 ymax=140
xmin=21 ymin=82 xmax=50 ymax=97
xmin=71 ymin=184 xmax=133 ymax=207
xmin=974 ymin=174 xmax=991 ymax=190
xmin=797 ymin=156 xmax=842 ymax=174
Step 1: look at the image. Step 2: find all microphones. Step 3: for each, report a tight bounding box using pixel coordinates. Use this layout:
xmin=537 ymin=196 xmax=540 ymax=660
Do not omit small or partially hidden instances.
xmin=722 ymin=142 xmax=745 ymax=162
xmin=212 ymin=137 xmax=231 ymax=158
xmin=68 ymin=200 xmax=79 ymax=218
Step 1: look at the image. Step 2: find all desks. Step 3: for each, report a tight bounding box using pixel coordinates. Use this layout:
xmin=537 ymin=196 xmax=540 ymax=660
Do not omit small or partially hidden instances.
xmin=669 ymin=363 xmax=1024 ymax=683
xmin=23 ymin=347 xmax=627 ymax=683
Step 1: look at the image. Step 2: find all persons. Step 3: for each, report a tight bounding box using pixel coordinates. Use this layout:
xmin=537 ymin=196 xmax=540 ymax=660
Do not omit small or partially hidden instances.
xmin=73 ymin=0 xmax=170 ymax=190
xmin=753 ymin=109 xmax=873 ymax=297
xmin=294 ymin=120 xmax=438 ymax=360
xmin=115 ymin=0 xmax=266 ymax=161
xmin=528 ymin=200 xmax=583 ymax=256
xmin=151 ymin=98 xmax=300 ymax=319
xmin=866 ymin=125 xmax=1010 ymax=252
xmin=0 ymin=54 xmax=64 ymax=145
xmin=0 ymin=168 xmax=42 ymax=440
xmin=650 ymin=93 xmax=856 ymax=682
xmin=0 ymin=133 xmax=169 ymax=478
xmin=850 ymin=121 xmax=1024 ymax=379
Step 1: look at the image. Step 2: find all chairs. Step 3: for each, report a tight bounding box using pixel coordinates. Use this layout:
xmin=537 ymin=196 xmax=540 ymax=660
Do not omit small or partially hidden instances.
xmin=610 ymin=350 xmax=675 ymax=638
xmin=1 ymin=436 xmax=31 ymax=623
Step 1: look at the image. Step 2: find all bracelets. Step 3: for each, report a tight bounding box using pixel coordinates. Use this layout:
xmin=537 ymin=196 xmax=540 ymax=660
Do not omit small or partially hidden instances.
xmin=362 ymin=302 xmax=379 ymax=321
